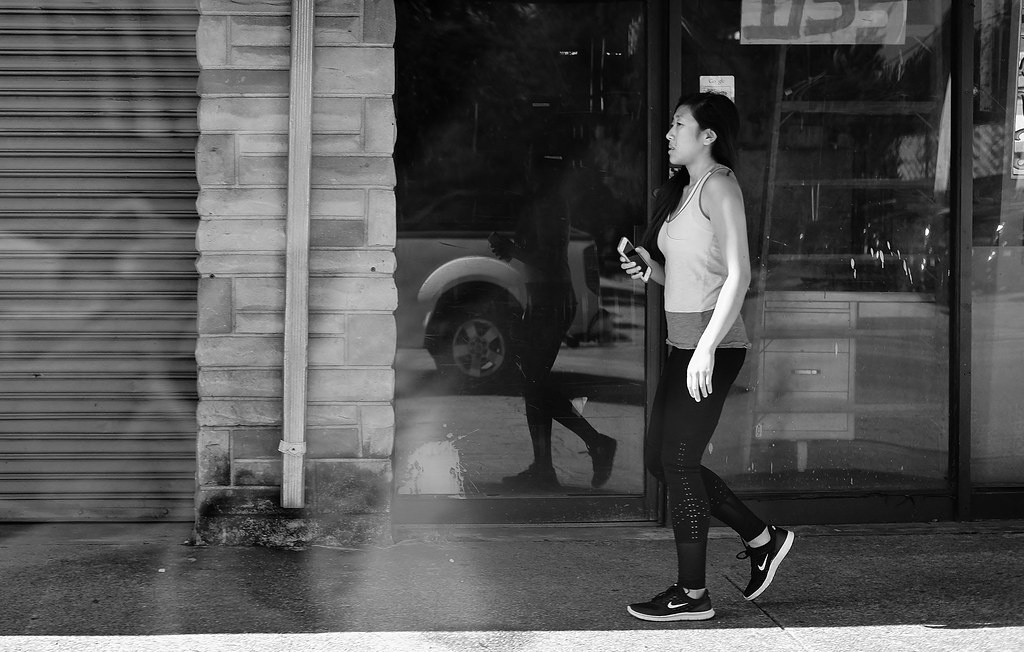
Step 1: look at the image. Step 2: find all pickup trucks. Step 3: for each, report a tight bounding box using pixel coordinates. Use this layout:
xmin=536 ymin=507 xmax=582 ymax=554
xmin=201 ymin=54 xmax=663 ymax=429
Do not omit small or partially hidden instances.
xmin=390 ymin=232 xmax=609 ymax=394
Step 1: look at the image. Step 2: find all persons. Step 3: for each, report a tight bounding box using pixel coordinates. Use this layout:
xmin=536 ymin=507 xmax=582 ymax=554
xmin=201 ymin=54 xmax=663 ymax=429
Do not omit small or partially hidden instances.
xmin=490 ymin=133 xmax=617 ymax=490
xmin=620 ymin=92 xmax=795 ymax=621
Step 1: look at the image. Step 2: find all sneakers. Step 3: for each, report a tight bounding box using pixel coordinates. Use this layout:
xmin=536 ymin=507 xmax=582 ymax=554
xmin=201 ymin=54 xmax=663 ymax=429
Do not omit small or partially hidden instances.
xmin=502 ymin=463 xmax=560 ymax=490
xmin=626 ymin=585 xmax=715 ymax=622
xmin=585 ymin=433 xmax=616 ymax=487
xmin=736 ymin=525 xmax=794 ymax=600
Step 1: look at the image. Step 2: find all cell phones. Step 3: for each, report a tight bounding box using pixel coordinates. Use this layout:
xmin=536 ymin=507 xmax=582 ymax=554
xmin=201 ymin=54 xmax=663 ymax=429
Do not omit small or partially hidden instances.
xmin=617 ymin=237 xmax=652 ymax=283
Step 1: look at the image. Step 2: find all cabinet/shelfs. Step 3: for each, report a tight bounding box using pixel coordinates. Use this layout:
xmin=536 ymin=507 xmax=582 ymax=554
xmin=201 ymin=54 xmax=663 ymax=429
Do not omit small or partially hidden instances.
xmin=759 ymin=42 xmax=971 ymax=294
xmin=760 ymin=288 xmax=857 ymax=469
